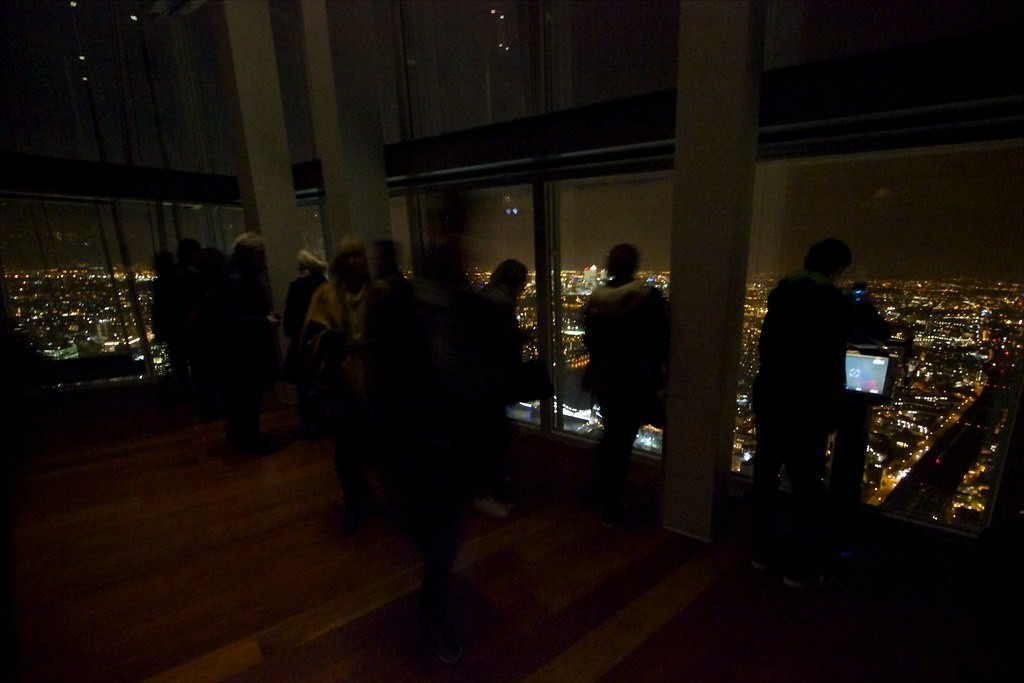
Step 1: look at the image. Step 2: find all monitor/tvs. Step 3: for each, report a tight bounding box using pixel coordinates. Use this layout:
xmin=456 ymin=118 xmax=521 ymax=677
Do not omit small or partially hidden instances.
xmin=842 ymin=345 xmax=899 ymax=405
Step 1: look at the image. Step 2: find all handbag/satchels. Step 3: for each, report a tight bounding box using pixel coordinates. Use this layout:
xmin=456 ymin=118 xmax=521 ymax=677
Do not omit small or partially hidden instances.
xmin=520 ymin=341 xmax=554 ymax=404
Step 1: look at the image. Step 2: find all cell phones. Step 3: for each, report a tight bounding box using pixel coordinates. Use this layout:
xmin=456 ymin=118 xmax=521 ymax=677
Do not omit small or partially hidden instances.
xmin=850 ymin=284 xmax=866 ymax=303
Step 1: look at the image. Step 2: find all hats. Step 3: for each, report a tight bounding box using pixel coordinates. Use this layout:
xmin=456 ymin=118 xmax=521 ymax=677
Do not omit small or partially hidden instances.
xmin=298 ymin=249 xmax=329 ymax=275
xmin=233 ymin=231 xmax=265 ymax=253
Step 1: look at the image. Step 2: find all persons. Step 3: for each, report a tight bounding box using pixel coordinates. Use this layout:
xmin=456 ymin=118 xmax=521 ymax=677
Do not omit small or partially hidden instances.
xmin=149 ymin=233 xmax=553 ymax=541
xmin=582 ymin=243 xmax=672 ymax=514
xmin=736 ymin=235 xmax=892 ymax=530
xmin=389 ymin=190 xmax=506 ymax=665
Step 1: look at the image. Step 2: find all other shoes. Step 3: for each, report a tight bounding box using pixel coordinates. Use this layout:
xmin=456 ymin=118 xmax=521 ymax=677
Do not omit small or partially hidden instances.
xmin=426 ymin=620 xmax=461 ymax=664
xmin=600 ymin=503 xmax=619 ymax=528
xmin=233 ymin=433 xmax=279 ymax=456
xmin=292 ymin=423 xmax=328 ymax=440
